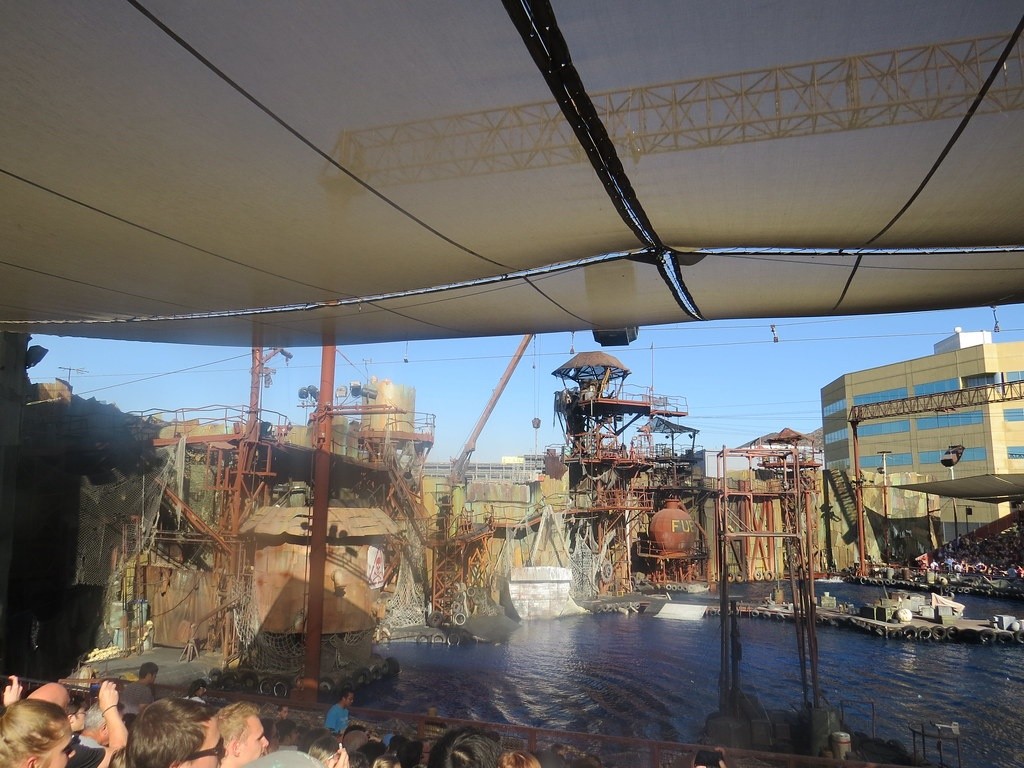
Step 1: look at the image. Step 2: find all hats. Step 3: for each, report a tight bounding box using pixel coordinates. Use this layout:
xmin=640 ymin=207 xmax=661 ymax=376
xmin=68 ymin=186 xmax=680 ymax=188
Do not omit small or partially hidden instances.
xmin=343 ymin=730 xmax=369 ymax=753
xmin=382 ymin=734 xmax=395 ymax=747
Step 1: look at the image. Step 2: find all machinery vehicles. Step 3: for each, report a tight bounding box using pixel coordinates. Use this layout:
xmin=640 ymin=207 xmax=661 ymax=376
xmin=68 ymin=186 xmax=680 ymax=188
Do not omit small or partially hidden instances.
xmin=448 ymin=335 xmax=542 ymax=483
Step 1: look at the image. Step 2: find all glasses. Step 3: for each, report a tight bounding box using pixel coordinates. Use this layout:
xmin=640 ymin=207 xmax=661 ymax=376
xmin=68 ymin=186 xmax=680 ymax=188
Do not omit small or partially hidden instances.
xmin=75 ymin=710 xmax=88 ymax=716
xmin=184 ymin=735 xmax=225 ymax=760
xmin=200 ymin=687 xmax=207 ymax=692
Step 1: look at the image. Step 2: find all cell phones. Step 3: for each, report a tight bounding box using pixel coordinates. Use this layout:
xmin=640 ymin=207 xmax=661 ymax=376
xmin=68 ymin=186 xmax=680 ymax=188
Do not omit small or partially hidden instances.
xmin=693 ymin=750 xmax=724 ymax=768
xmin=338 ymin=743 xmax=342 ymax=749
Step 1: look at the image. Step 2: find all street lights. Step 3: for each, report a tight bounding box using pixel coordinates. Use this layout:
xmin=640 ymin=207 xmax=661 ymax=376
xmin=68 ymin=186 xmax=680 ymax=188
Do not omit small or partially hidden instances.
xmin=878 ymin=450 xmax=894 ymax=558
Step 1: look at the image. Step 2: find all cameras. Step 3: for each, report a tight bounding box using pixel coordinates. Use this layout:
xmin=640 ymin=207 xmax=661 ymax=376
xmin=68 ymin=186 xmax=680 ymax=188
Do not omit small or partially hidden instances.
xmin=90 ymin=683 xmax=101 ymax=699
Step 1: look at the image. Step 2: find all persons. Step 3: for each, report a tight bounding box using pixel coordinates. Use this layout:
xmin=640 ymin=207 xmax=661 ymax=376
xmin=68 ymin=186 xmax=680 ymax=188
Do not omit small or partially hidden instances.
xmin=930 ymin=518 xmax=1024 ymax=577
xmin=0 ymin=662 xmax=733 ymax=768
